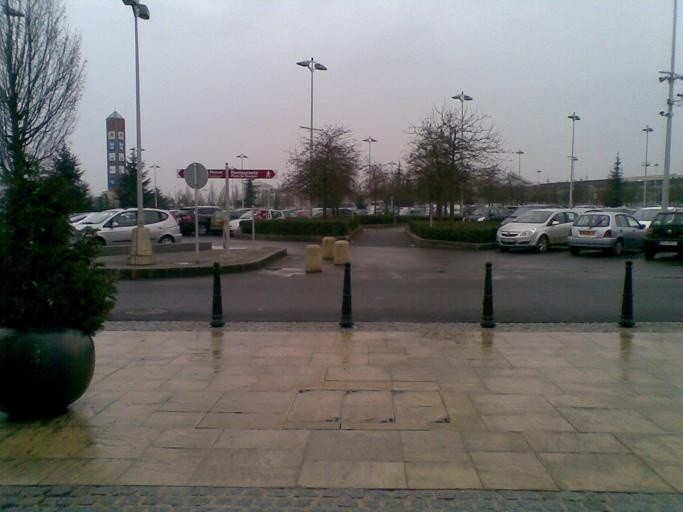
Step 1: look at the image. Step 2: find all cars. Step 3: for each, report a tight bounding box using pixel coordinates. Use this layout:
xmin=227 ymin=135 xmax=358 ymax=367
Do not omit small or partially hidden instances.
xmin=567 ymin=210 xmax=647 ymax=256
xmin=407 ymin=203 xmax=683 ymax=231
xmin=640 ymin=208 xmax=683 ymax=262
xmin=69 ymin=211 xmax=99 ymax=223
xmin=495 ymin=208 xmax=581 ymax=254
xmin=167 ymin=203 xmax=384 ymax=238
xmin=69 ymin=206 xmax=183 ymax=248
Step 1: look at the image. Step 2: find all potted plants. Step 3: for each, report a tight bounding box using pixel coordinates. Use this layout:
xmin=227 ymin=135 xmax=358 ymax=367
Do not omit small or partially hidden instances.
xmin=1 ymin=144 xmax=121 ymax=421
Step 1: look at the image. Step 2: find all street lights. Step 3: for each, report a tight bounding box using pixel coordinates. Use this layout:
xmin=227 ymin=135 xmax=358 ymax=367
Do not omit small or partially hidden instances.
xmin=450 ymin=89 xmax=474 ymax=216
xmin=120 ymin=0 xmax=157 ymax=266
xmin=514 ymin=148 xmax=524 ymax=177
xmin=234 ymin=151 xmax=249 ymax=209
xmin=362 ymin=134 xmax=378 ymax=177
xmin=565 ymin=110 xmax=580 ymax=207
xmin=294 ymin=56 xmax=328 ymax=218
xmin=536 ymin=168 xmax=542 ymax=191
xmin=639 ymin=122 xmax=654 ymax=207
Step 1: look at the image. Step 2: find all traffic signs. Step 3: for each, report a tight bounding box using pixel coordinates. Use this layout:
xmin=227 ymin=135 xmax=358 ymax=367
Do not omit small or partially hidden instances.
xmin=176 ymin=167 xmax=226 ymax=180
xmin=227 ymin=168 xmax=276 ymax=179
